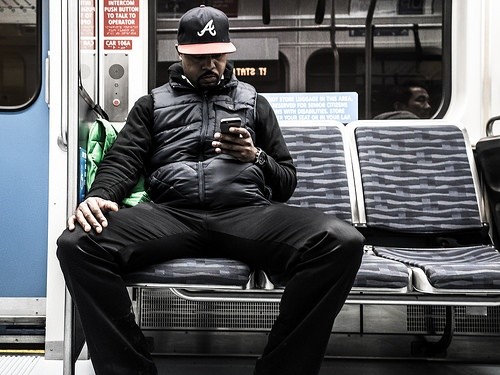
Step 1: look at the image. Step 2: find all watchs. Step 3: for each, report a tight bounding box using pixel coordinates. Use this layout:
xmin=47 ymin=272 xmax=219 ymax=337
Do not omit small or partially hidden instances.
xmin=253 ymin=147 xmax=266 ymax=165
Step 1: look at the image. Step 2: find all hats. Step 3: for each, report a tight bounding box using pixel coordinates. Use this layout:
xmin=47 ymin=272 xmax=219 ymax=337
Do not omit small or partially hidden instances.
xmin=176 ymin=4 xmax=237 ymax=55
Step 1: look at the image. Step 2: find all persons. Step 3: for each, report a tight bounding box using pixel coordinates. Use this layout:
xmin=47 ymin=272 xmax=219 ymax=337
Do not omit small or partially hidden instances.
xmin=55 ymin=5 xmax=366 ymax=375
xmin=372 ymin=85 xmax=432 ymax=120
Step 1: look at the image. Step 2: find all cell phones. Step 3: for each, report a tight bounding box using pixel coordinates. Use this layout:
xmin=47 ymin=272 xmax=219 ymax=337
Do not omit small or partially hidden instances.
xmin=220 ymin=118 xmax=241 ymax=145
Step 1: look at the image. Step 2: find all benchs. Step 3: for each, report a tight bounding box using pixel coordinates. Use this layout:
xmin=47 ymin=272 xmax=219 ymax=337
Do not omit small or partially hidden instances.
xmin=127 ymin=119 xmax=500 ymax=356
